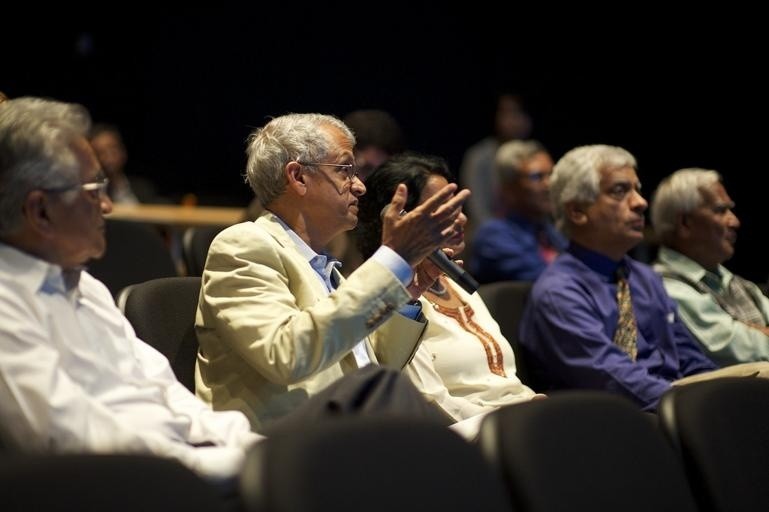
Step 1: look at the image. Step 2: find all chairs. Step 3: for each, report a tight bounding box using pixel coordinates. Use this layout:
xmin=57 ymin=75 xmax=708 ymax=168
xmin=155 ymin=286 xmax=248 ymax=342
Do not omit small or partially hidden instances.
xmin=474 ymin=280 xmax=564 ymax=394
xmin=0 ymin=446 xmax=229 ymax=512
xmin=477 ymin=387 xmax=702 ymax=512
xmin=114 ymin=276 xmax=203 ymax=395
xmin=657 ymin=360 xmax=769 ymax=512
xmin=234 ymin=408 xmax=498 ymax=512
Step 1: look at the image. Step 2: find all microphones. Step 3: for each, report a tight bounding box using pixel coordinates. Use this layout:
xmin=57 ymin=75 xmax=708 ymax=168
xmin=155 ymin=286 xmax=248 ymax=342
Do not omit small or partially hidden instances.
xmin=380 ymin=202 xmax=482 ymax=295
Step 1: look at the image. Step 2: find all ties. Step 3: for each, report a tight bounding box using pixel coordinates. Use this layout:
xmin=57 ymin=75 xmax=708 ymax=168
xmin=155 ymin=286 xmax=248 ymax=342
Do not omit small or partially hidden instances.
xmin=616 ymin=262 xmax=638 ymax=362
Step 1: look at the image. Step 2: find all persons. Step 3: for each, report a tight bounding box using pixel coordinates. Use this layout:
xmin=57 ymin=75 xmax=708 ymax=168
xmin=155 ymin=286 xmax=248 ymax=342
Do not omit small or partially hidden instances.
xmin=463 ymin=92 xmax=537 ymax=235
xmin=518 ymin=140 xmax=722 ymax=423
xmin=341 ymin=110 xmax=409 ymax=169
xmin=469 ymin=138 xmax=557 ymax=285
xmin=0 ymin=95 xmax=269 ymax=481
xmin=88 ymin=118 xmax=158 ymax=212
xmin=648 ymin=164 xmax=769 ymax=363
xmin=355 ymin=155 xmax=544 ymax=432
xmin=193 ymin=106 xmax=472 ymax=435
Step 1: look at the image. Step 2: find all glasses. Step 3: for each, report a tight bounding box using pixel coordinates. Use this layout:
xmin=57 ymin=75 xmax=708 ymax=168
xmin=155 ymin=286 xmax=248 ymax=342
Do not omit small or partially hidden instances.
xmin=298 ymin=161 xmax=357 ymax=178
xmin=44 ymin=176 xmax=109 ymax=193
